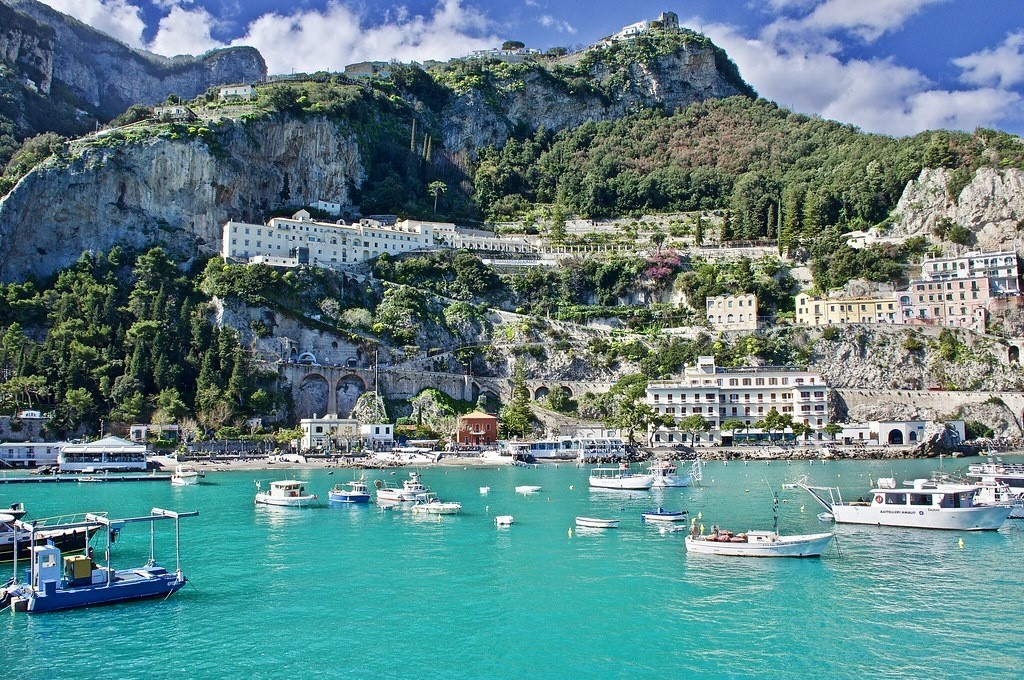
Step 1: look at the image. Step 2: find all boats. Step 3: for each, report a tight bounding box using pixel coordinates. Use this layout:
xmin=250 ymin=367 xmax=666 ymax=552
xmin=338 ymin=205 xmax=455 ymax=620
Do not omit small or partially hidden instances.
xmin=254 ymin=479 xmax=316 ymax=506
xmin=953 ymin=442 xmax=1024 ymax=499
xmin=684 ymin=491 xmax=837 ymax=559
xmin=902 ymin=454 xmax=1024 ymax=519
xmin=632 ymin=455 xmax=704 ymax=487
xmin=328 ymin=481 xmax=373 ymax=504
xmin=171 ymin=464 xmax=205 ymax=485
xmin=493 ymin=515 xmax=513 ymax=524
xmin=78 ymin=478 xmax=104 ymax=482
xmin=410 ymin=493 xmax=462 ymax=514
xmin=792 ymin=472 xmax=1015 ymax=531
xmin=515 ymin=486 xmax=542 ymax=493
xmin=0 ymin=507 xmax=200 ymax=615
xmin=782 ymin=483 xmax=797 ymax=489
xmin=375 ymin=471 xmax=431 ymax=502
xmin=641 ymin=507 xmax=689 ymax=522
xmin=0 ymin=503 xmax=108 ymax=563
xmin=588 ymin=455 xmax=657 ymax=489
xmin=479 ymin=486 xmax=490 ymax=496
xmin=576 ymin=517 xmax=622 ymax=528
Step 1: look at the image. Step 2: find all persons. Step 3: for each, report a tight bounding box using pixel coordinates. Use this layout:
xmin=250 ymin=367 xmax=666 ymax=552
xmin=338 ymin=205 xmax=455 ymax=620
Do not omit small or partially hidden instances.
xmin=865 ymin=498 xmax=870 ymax=506
xmin=89 ymin=547 xmax=98 ymax=569
xmin=445 ymin=445 xmax=458 ymax=452
xmin=857 ymin=497 xmax=863 ymax=506
xmin=332 ymin=455 xmax=355 ymax=465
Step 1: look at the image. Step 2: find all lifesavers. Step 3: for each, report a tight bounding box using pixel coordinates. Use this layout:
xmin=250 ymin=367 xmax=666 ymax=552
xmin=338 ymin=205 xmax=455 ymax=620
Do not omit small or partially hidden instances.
xmin=874 ymin=495 xmax=884 ymax=503
